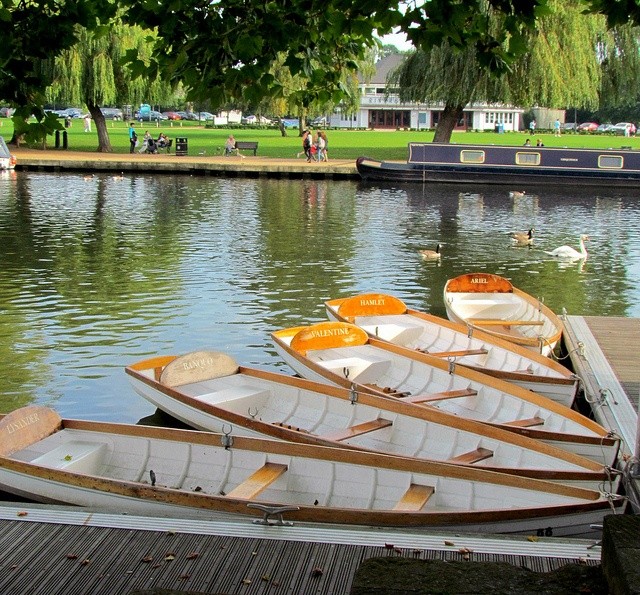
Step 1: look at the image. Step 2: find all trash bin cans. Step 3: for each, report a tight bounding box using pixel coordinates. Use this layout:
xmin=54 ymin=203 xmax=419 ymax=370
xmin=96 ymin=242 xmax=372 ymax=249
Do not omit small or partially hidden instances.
xmin=176 ymin=137 xmax=187 ymax=155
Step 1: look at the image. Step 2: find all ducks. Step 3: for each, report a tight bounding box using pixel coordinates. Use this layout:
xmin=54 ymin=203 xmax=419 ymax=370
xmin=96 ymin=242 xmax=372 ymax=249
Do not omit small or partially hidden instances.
xmin=507 ymin=228 xmax=536 ymax=243
xmin=417 ymin=244 xmax=445 ymax=261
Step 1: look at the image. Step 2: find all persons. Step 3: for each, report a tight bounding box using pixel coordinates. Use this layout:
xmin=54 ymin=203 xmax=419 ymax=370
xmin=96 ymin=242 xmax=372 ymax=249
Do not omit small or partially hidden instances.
xmin=296 ymin=128 xmax=330 ymax=162
xmin=138 ymin=129 xmax=170 ymax=153
xmin=129 ymin=123 xmax=138 ymax=154
xmin=554 ymin=118 xmax=561 ymax=137
xmin=530 ymin=119 xmax=536 ymax=136
xmin=522 ymin=138 xmax=532 ymax=146
xmin=225 ymin=135 xmax=235 ymax=157
xmin=66 ymin=114 xmax=91 ymax=133
xmin=536 ymin=139 xmax=545 ymax=146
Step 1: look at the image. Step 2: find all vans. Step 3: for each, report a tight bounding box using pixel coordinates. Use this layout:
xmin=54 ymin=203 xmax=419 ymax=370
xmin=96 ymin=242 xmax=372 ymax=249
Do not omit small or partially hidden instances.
xmin=100 ymin=108 xmax=122 ymax=120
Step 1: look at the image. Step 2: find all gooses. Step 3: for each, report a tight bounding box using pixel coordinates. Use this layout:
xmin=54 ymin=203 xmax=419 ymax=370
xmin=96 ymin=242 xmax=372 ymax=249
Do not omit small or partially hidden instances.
xmin=543 ymin=234 xmax=591 ymax=259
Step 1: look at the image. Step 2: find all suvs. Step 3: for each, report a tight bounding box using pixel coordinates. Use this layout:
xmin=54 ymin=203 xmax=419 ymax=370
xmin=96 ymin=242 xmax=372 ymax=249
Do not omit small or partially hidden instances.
xmin=612 ymin=123 xmax=635 ymax=134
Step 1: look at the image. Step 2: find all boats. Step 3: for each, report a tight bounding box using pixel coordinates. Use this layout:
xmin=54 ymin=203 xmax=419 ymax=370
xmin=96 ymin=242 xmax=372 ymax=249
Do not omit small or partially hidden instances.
xmin=124 ymin=351 xmax=623 ymax=495
xmin=269 ymin=322 xmax=621 ymax=471
xmin=444 ymin=272 xmax=564 ymax=358
xmin=356 ymin=142 xmax=640 ymax=187
xmin=0 ymin=404 xmax=628 ymax=537
xmin=324 ymin=293 xmax=578 ymax=409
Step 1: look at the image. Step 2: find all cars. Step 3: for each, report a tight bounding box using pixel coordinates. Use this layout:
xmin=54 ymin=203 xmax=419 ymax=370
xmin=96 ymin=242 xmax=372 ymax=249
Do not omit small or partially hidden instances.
xmin=200 ymin=112 xmax=216 ymax=120
xmin=138 ymin=110 xmax=168 ymax=122
xmin=181 ymin=115 xmax=190 ymax=120
xmin=54 ymin=108 xmax=76 ymax=119
xmin=576 ymin=122 xmax=599 ymax=132
xmin=242 ymin=115 xmax=272 ymax=125
xmin=561 ymin=123 xmax=575 ymax=130
xmin=168 ymin=112 xmax=181 ymax=120
xmin=597 ymin=124 xmax=614 ymax=133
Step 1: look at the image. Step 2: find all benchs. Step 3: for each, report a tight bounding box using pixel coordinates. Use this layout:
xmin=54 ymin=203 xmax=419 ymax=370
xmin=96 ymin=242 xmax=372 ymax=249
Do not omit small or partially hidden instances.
xmin=235 ymin=142 xmax=258 ymax=156
xmin=153 ymin=139 xmax=172 ymax=154
